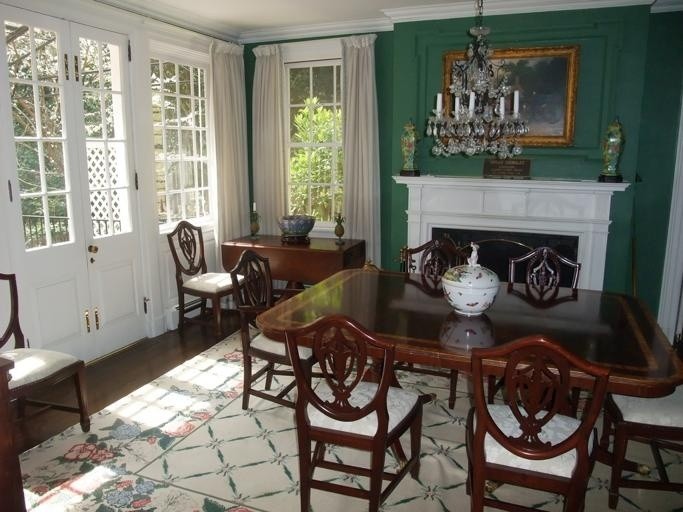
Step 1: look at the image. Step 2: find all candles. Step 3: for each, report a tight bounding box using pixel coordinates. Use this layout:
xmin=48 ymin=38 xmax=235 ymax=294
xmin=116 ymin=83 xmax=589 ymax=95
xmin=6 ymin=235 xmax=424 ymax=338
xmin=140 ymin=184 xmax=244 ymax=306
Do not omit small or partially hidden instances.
xmin=336 ymin=202 xmax=341 ymax=213
xmin=252 ymin=201 xmax=257 ymax=211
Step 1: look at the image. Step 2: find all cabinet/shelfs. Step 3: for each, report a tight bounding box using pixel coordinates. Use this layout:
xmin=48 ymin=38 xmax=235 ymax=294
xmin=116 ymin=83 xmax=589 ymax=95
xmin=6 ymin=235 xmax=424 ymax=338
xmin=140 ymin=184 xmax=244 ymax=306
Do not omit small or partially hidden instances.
xmin=0 ymin=357 xmax=29 ymax=512
xmin=392 ymin=173 xmax=632 ymax=292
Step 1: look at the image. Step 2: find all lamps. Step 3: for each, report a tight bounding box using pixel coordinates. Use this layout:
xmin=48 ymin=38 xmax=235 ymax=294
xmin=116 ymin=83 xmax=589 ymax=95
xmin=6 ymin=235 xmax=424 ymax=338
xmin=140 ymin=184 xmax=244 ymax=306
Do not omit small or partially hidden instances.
xmin=425 ymin=0 xmax=530 ymax=161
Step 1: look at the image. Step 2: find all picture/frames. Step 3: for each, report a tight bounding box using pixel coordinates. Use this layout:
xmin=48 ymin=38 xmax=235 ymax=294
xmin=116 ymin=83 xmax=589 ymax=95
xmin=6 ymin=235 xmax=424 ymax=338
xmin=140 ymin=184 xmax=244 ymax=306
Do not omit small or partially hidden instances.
xmin=443 ymin=46 xmax=579 ymax=148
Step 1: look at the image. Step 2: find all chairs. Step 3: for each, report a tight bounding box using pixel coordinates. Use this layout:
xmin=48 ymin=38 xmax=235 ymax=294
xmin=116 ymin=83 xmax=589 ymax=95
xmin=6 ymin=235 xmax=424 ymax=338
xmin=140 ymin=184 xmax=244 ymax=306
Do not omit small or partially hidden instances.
xmin=0 ymin=272 xmax=90 ymax=435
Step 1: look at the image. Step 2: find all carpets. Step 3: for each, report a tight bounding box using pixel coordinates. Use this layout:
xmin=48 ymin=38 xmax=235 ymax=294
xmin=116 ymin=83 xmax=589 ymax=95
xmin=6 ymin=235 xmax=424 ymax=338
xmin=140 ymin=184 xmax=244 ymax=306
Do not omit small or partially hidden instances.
xmin=16 ymin=321 xmax=683 ymax=512
